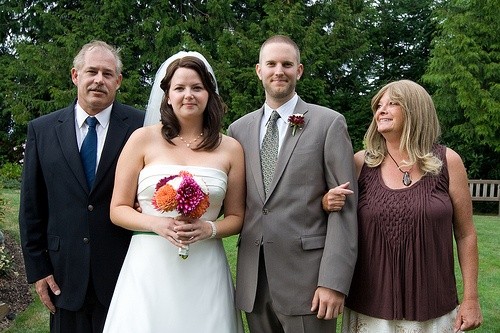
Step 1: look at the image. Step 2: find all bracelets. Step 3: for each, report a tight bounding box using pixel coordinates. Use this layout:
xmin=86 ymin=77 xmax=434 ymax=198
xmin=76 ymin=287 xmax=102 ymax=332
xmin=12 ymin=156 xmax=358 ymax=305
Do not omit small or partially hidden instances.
xmin=205 ymin=220 xmax=216 ymax=239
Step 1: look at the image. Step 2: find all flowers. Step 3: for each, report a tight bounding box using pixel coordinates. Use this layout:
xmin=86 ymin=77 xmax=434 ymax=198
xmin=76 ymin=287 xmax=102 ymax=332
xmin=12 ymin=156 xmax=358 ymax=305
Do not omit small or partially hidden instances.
xmin=287 ymin=113 xmax=306 ymax=135
xmin=152 ymin=171 xmax=209 ymax=261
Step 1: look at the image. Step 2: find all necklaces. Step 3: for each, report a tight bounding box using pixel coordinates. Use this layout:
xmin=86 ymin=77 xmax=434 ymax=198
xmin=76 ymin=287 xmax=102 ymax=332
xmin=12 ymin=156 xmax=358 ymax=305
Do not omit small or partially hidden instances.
xmin=387 ymin=150 xmax=411 ymax=186
xmin=178 ymin=133 xmax=203 ymax=147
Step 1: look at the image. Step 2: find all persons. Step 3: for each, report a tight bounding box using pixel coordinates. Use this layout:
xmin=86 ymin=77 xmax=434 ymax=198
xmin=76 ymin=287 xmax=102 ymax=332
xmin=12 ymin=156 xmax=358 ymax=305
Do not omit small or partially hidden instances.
xmin=18 ymin=41 xmax=146 ymax=333
xmin=104 ymin=51 xmax=245 ymax=333
xmin=134 ymin=34 xmax=358 ymax=333
xmin=323 ymin=80 xmax=482 ymax=333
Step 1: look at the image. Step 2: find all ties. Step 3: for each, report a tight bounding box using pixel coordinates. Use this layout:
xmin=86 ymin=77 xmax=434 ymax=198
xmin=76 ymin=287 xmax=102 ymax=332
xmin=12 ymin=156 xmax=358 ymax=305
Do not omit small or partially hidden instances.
xmin=260 ymin=109 xmax=280 ymax=195
xmin=79 ymin=116 xmax=97 ymax=191
